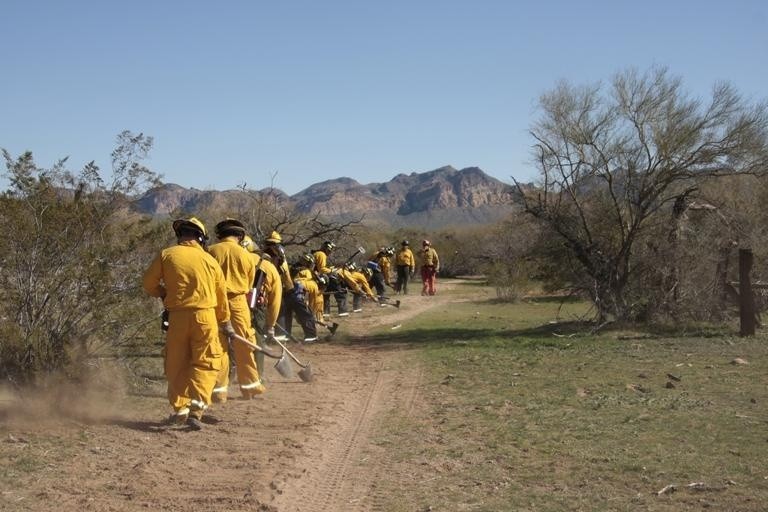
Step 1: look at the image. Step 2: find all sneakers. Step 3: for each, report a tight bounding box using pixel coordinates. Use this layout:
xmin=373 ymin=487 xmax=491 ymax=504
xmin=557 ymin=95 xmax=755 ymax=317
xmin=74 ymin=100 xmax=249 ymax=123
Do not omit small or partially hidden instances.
xmin=187 ymin=416 xmax=202 ymax=430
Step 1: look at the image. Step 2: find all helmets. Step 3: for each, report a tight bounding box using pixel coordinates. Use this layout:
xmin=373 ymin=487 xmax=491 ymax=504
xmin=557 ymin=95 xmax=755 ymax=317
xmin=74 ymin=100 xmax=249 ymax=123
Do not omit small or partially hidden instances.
xmin=173 ymin=215 xmax=282 ymax=244
xmin=300 ymin=241 xmax=336 ymax=289
xmin=343 ymin=240 xmax=431 ymax=281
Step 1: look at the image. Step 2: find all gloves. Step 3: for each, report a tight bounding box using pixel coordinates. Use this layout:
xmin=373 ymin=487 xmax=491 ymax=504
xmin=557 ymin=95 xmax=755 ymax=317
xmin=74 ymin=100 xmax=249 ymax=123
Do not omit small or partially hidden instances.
xmin=222 ymin=321 xmax=235 ymax=339
xmin=264 ymin=327 xmax=276 ymax=346
xmin=359 ymin=290 xmax=367 ymax=298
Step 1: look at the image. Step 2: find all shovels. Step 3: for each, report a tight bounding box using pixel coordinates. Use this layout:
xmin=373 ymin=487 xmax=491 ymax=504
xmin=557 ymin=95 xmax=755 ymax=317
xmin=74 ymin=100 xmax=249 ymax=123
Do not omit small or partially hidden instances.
xmin=218 ymin=327 xmax=292 ymax=379
xmin=255 ymin=308 xmax=305 ymax=352
xmin=251 ymin=323 xmax=313 ymax=382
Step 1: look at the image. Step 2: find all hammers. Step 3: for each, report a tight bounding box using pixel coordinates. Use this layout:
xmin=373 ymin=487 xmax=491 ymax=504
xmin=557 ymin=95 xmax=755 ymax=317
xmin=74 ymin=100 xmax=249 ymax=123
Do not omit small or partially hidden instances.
xmin=378 ymin=300 xmax=400 ymax=307
xmin=344 ymin=246 xmax=365 ymax=264
xmin=315 ymin=321 xmax=339 ymax=336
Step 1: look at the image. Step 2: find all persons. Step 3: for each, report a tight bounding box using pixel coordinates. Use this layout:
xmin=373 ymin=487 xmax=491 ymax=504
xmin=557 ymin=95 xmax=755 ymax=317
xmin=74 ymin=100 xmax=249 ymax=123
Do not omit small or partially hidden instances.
xmin=206 ymin=216 xmax=265 ymax=406
xmin=139 ymin=213 xmax=223 ymax=432
xmin=394 ymin=238 xmax=415 ymax=297
xmin=416 ymin=239 xmax=441 ymax=297
xmin=227 ymin=228 xmax=395 ymax=395
